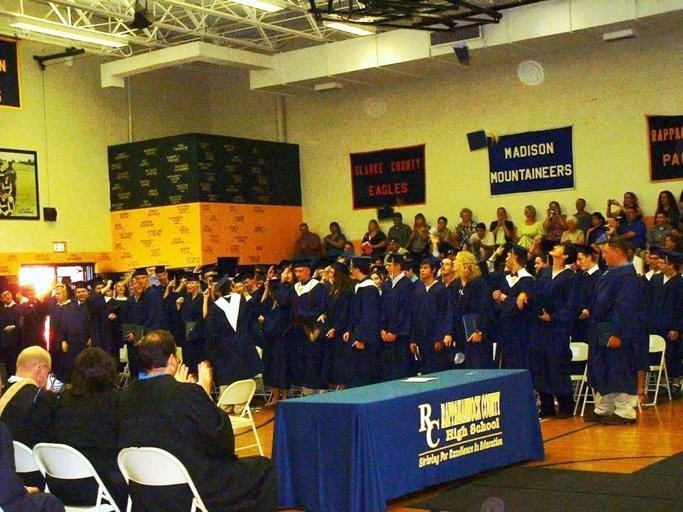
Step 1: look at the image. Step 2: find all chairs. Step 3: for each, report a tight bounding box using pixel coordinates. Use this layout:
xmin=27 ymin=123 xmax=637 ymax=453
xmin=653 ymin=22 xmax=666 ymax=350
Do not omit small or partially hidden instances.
xmin=253 ymin=345 xmax=268 ymax=402
xmin=33 ymin=443 xmax=122 ymax=512
xmin=116 ymin=445 xmax=208 ymax=512
xmin=569 ymin=342 xmax=589 ymax=415
xmin=635 ymin=335 xmax=672 ymax=406
xmin=12 ymin=440 xmax=50 ymax=491
xmin=216 ymin=379 xmax=264 ymax=457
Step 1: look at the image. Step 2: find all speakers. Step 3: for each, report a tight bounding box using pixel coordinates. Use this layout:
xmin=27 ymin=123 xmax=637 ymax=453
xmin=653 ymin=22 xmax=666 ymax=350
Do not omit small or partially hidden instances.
xmin=467 ymin=129 xmax=488 ymax=151
xmin=44 ymin=208 xmax=57 ymax=221
xmin=454 ymin=42 xmax=471 ymax=66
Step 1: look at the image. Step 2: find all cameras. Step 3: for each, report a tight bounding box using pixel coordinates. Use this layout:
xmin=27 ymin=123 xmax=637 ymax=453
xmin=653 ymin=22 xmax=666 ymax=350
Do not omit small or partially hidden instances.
xmin=549 ymin=208 xmax=555 ymax=213
xmin=610 ymin=200 xmax=613 ymax=203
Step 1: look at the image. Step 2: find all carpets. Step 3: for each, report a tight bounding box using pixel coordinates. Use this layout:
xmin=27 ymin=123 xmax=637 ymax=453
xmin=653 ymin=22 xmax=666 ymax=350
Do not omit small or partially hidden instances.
xmin=408 ymin=451 xmax=683 ymax=510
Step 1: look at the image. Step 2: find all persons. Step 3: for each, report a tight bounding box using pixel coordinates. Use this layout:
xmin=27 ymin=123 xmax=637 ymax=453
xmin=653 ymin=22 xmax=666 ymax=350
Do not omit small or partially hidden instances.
xmin=0 ymin=420 xmax=66 ymax=512
xmin=0 ymin=189 xmax=683 ymax=422
xmin=45 ymin=347 xmax=137 ymax=512
xmin=116 ymin=328 xmax=278 ymax=511
xmin=0 ymin=344 xmax=59 ymax=493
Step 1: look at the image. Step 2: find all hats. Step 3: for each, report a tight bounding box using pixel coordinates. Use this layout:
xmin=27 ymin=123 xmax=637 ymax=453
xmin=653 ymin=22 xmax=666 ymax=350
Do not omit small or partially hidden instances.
xmin=275 ymin=259 xmax=291 ymax=274
xmin=133 ymin=265 xmax=150 ymax=275
xmin=183 ymin=271 xmax=199 ymax=280
xmin=502 ymin=243 xmax=530 ymax=262
xmin=426 ymin=254 xmax=444 ymax=270
xmin=215 ymin=273 xmax=231 ymax=292
xmin=574 ymin=243 xmax=599 ymax=256
xmin=348 ymin=255 xmax=372 ymax=269
xmin=107 ymin=272 xmax=124 ymax=290
xmin=86 ymin=277 xmax=103 ymax=286
xmin=166 ymin=266 xmax=184 ymax=278
xmin=291 ymin=255 xmax=317 ymax=267
xmin=150 ymin=263 xmax=169 ymax=273
xmin=657 ymin=248 xmax=683 ymax=264
xmin=384 ymin=250 xmax=404 ymax=263
xmin=648 ymin=244 xmax=663 ymax=254
xmin=199 ymin=263 xmax=217 ymax=273
xmin=330 ymin=262 xmax=350 ymax=276
xmin=70 ymin=280 xmax=87 ymax=289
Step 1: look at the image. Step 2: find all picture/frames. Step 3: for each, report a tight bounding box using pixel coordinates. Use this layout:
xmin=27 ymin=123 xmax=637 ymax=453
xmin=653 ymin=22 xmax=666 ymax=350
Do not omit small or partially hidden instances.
xmin=0 ymin=147 xmax=41 ymax=220
xmin=1 ymin=35 xmax=23 ymax=110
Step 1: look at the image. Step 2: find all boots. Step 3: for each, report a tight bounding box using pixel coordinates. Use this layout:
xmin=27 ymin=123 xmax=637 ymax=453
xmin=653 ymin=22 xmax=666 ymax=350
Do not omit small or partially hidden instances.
xmin=538 ymin=391 xmax=557 ymax=418
xmin=557 ymin=393 xmax=574 ymax=419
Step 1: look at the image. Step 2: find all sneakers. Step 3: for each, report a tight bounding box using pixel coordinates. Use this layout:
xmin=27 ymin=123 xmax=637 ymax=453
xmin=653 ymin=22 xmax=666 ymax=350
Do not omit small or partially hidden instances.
xmin=670 ymin=384 xmax=682 ymax=398
xmin=656 ymin=386 xmax=668 ymax=397
xmin=603 ymin=412 xmax=638 ymax=426
xmin=583 ymin=411 xmax=608 ymax=423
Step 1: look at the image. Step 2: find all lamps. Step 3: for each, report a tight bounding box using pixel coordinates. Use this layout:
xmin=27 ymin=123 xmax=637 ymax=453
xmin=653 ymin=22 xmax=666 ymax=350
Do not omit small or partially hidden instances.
xmin=322 ymin=17 xmax=375 ymax=36
xmin=228 ymin=1 xmax=286 ymax=14
xmin=8 ymin=15 xmax=128 ymax=48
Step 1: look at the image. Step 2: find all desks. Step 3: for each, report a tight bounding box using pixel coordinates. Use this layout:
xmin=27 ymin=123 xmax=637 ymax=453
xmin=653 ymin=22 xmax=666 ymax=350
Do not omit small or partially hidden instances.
xmin=273 ymin=368 xmax=545 ymax=511
